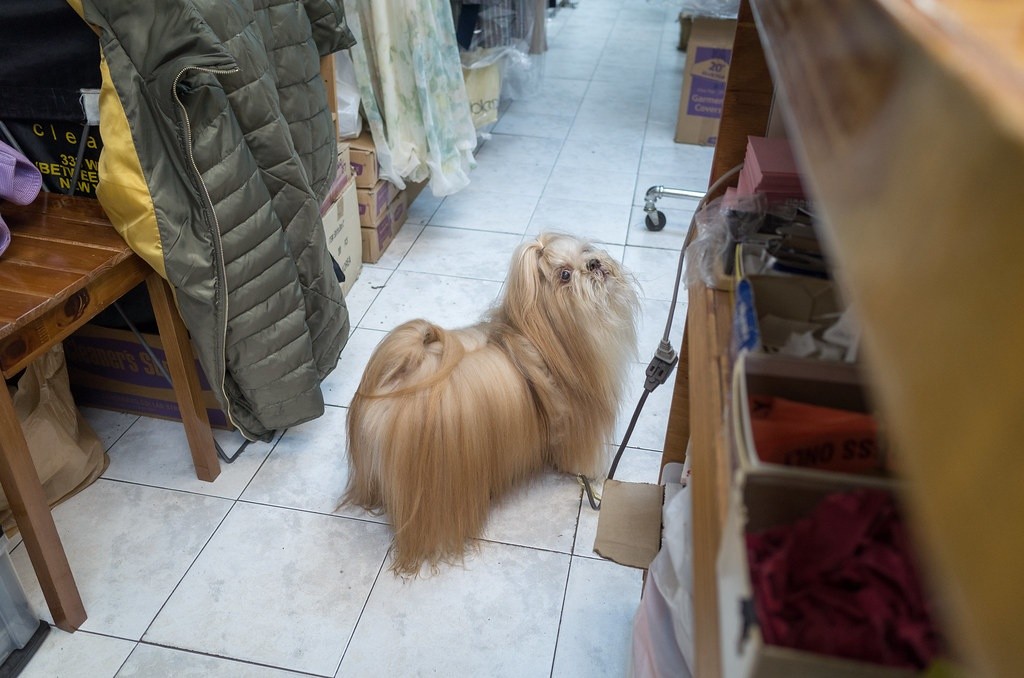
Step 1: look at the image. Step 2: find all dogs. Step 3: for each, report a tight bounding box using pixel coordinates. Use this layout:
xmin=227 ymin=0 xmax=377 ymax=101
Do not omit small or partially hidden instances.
xmin=334 ymin=231 xmax=645 ymax=580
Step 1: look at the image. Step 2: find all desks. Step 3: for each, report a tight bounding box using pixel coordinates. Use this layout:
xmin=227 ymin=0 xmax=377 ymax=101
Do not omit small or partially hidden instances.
xmin=1 ymin=194 xmax=219 ymax=630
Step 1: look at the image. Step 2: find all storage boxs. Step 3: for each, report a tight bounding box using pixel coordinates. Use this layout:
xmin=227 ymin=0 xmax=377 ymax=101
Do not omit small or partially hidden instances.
xmin=673 ymin=13 xmax=738 ymax=145
xmin=60 ymin=324 xmax=238 ymax=433
xmin=317 ymin=129 xmax=431 ymax=298
xmin=596 ymin=136 xmax=957 ymax=678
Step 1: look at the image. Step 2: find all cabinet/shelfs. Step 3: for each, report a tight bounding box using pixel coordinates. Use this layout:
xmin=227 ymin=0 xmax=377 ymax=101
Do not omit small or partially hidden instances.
xmin=653 ymin=4 xmax=1022 ymax=677
